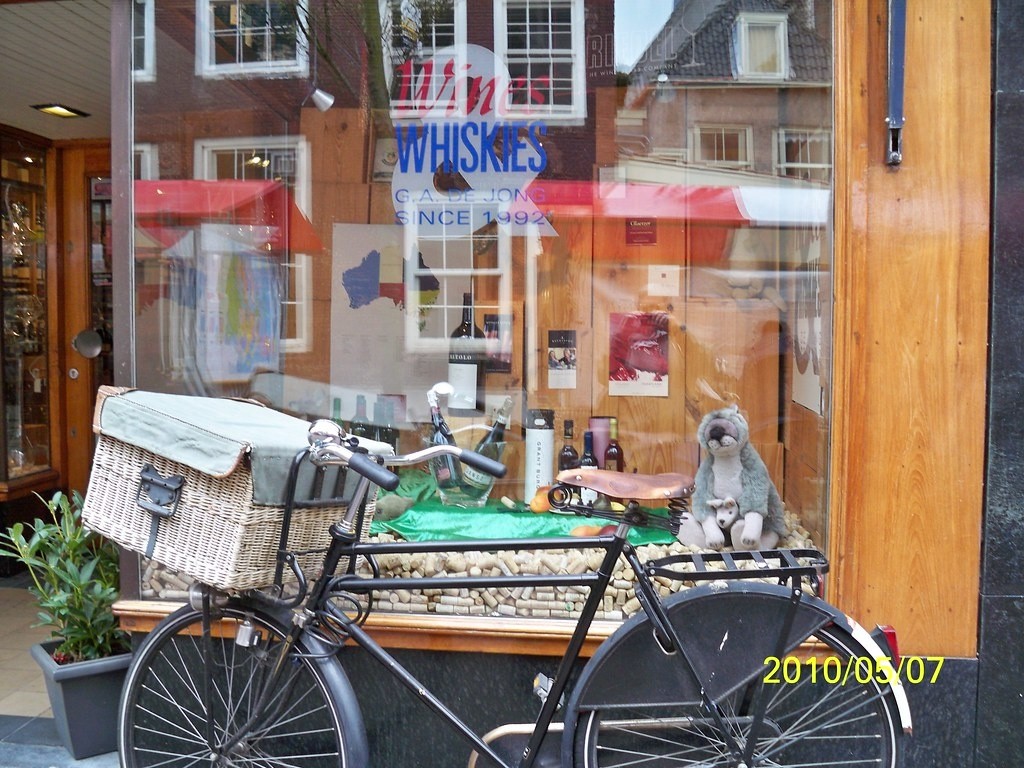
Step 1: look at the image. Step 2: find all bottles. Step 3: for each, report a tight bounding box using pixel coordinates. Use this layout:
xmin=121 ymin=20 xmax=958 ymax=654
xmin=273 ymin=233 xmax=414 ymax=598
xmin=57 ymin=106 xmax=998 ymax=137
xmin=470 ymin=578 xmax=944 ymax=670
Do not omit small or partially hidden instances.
xmin=457 ymin=399 xmax=516 ymax=496
xmin=351 ymin=394 xmax=401 ymax=476
xmin=447 ymin=291 xmax=486 ymax=418
xmin=604 ymin=419 xmax=624 ymax=506
xmin=331 ymin=398 xmax=344 ymax=430
xmin=558 ymin=420 xmax=580 ymax=506
xmin=577 ymin=433 xmax=600 ymax=507
xmin=426 ymin=390 xmax=462 ymax=489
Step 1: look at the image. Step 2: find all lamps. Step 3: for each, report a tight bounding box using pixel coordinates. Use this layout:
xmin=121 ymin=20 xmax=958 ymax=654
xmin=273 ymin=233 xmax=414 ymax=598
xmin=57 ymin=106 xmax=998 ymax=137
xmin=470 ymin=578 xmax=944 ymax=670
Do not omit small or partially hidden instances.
xmin=302 ymin=9 xmax=335 ymax=112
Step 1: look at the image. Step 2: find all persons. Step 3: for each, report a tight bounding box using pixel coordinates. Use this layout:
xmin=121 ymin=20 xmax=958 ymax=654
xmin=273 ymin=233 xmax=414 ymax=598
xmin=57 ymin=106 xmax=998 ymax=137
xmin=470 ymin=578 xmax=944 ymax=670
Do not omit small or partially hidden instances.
xmin=548 ymin=348 xmax=573 ymax=369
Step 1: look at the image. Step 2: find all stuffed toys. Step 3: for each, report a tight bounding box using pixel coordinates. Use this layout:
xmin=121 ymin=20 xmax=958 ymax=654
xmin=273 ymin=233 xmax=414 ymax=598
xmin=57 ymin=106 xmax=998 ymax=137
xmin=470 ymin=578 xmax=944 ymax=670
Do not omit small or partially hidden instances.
xmin=668 ymin=404 xmax=789 ymax=552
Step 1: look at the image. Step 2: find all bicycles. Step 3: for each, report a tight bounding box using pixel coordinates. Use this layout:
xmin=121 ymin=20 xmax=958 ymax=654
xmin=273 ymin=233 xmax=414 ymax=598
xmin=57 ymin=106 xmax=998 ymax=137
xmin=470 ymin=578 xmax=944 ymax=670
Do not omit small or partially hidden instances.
xmin=114 ymin=418 xmax=914 ymax=768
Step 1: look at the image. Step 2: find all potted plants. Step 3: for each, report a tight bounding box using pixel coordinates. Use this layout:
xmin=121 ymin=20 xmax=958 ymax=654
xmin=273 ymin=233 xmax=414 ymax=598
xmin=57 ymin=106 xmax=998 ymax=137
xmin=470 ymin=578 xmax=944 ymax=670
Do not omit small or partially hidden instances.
xmin=0 ymin=490 xmax=134 ymax=760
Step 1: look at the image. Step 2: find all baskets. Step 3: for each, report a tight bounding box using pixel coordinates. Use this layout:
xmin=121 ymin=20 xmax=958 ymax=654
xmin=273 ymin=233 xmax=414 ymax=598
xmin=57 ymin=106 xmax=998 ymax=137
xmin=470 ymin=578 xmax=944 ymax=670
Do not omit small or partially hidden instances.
xmin=79 ymin=433 xmax=379 ymax=590
